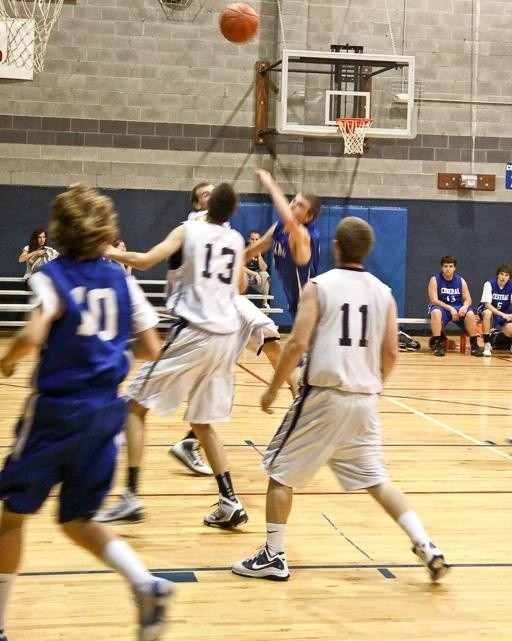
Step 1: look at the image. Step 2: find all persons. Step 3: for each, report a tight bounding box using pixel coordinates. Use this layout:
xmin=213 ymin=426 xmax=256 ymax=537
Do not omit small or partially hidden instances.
xmin=112 ymin=236 xmax=133 ymax=275
xmin=232 ymin=216 xmax=454 ymax=585
xmin=2 ymin=184 xmax=176 ymax=641
xmin=87 ymin=181 xmax=252 ymax=533
xmin=164 ymin=181 xmax=307 ymax=478
xmin=425 ymin=251 xmax=484 ymax=358
xmin=17 ymin=226 xmax=63 ymax=276
xmin=247 ymin=164 xmax=326 ymax=367
xmin=241 ymin=229 xmax=272 ymax=309
xmin=474 ymin=260 xmax=512 ymax=357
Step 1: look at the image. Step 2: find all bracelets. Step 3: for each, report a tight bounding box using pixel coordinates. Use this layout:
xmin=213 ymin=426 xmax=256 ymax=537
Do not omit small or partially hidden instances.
xmin=253 ymin=272 xmax=259 ymax=278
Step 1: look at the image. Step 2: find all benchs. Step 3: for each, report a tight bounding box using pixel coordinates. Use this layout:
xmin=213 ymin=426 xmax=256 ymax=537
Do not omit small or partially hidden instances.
xmin=396 ymin=317 xmax=455 ymax=325
xmin=0 ymin=275 xmax=285 ymax=329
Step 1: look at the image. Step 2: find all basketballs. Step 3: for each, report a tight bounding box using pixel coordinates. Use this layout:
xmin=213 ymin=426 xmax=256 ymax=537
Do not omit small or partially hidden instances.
xmin=220 ymin=2 xmax=260 ymax=42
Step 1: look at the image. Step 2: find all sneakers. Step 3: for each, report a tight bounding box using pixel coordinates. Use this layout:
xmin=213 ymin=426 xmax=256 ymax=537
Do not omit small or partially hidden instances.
xmin=482 ymin=342 xmax=493 ymax=356
xmin=168 ymin=437 xmax=213 ymax=475
xmin=231 ymin=542 xmax=289 ymax=580
xmin=412 ymin=537 xmax=450 ymax=580
xmin=132 ymin=579 xmax=176 ymax=641
xmin=471 ymin=347 xmax=482 ymax=356
xmin=91 ymin=489 xmax=146 ymax=522
xmin=434 ymin=345 xmax=444 ymax=356
xmin=203 ymin=492 xmax=249 ymax=529
